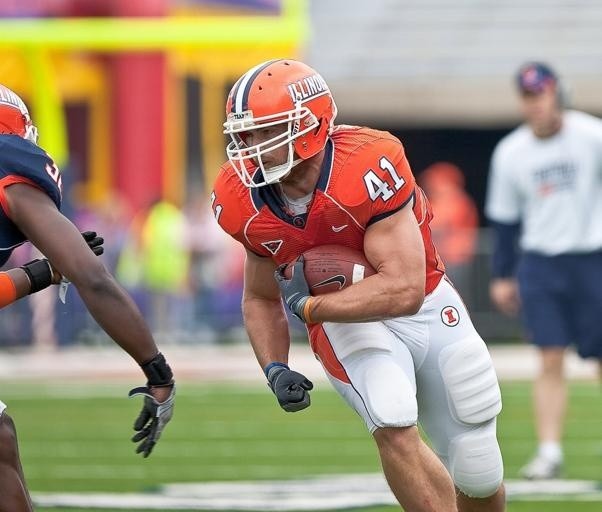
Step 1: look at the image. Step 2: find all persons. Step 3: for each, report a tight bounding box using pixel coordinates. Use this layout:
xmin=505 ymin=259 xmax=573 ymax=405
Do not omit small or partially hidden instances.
xmin=0 ymin=229 xmax=104 ymax=511
xmin=420 ymin=161 xmax=479 ymax=298
xmin=210 ymin=58 xmax=508 ymax=512
xmin=0 ymin=83 xmax=182 ymax=457
xmin=2 ymin=180 xmax=246 ymax=345
xmin=484 ymin=62 xmax=602 ymax=483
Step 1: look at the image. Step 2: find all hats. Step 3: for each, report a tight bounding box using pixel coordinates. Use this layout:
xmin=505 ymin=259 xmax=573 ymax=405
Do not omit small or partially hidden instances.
xmin=518 ymin=61 xmax=559 ymax=99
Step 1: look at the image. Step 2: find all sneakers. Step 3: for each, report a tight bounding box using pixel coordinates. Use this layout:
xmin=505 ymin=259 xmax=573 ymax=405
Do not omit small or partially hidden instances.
xmin=516 ymin=446 xmax=562 ymax=482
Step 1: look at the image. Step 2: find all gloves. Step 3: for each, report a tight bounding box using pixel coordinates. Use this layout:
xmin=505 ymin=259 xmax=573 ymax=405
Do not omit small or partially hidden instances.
xmin=81 ymin=228 xmax=105 ymax=259
xmin=128 ymin=381 xmax=177 ymax=459
xmin=267 ymin=367 xmax=315 ymax=414
xmin=272 ymin=253 xmax=312 ymax=322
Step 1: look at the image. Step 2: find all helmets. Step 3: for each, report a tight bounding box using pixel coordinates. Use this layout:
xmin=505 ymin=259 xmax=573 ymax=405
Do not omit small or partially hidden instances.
xmin=224 ymin=57 xmax=339 ymax=161
xmin=0 ymin=82 xmax=40 ymax=147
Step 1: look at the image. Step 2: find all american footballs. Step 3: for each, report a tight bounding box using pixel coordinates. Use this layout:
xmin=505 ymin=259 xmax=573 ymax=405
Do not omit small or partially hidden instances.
xmin=281 ymin=244 xmax=377 ymax=297
